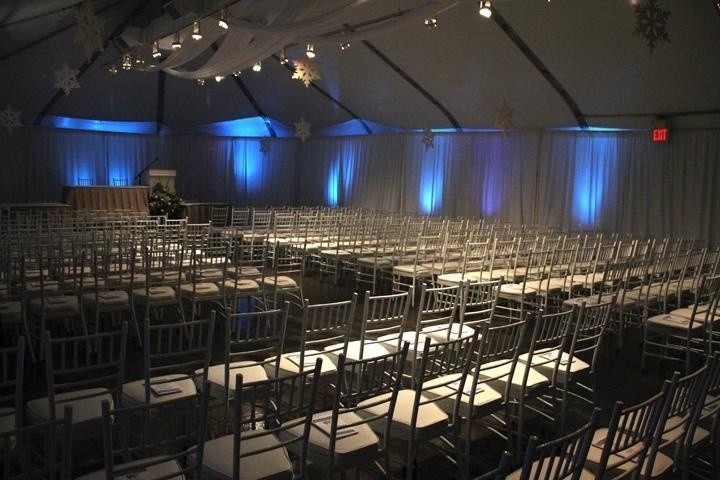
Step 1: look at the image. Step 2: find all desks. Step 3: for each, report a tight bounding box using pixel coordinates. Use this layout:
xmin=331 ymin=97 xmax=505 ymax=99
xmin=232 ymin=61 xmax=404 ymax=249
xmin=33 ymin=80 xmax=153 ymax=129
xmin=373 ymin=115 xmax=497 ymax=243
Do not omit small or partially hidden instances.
xmin=63 ymin=185 xmax=150 ymax=211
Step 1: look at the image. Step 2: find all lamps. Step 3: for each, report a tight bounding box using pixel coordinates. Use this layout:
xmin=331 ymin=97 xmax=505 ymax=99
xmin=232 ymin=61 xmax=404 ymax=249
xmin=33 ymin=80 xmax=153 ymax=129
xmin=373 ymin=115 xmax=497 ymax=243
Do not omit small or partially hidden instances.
xmin=99 ymin=0 xmax=249 ymax=74
xmin=196 ymin=0 xmax=493 ymax=88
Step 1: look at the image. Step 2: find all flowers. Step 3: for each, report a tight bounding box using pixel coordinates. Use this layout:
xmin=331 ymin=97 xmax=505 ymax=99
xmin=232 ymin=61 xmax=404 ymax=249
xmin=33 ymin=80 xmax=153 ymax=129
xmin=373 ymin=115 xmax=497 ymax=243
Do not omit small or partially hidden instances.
xmin=145 ymin=177 xmax=185 ymax=219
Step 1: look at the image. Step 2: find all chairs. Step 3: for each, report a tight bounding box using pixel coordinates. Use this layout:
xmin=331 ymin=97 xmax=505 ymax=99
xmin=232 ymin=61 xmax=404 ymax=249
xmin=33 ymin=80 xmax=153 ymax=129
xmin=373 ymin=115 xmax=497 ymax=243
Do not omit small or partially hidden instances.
xmin=0 ymin=203 xmax=720 ymax=480
xmin=113 ymin=178 xmax=128 ymax=186
xmin=78 ymin=176 xmax=93 ymax=185
